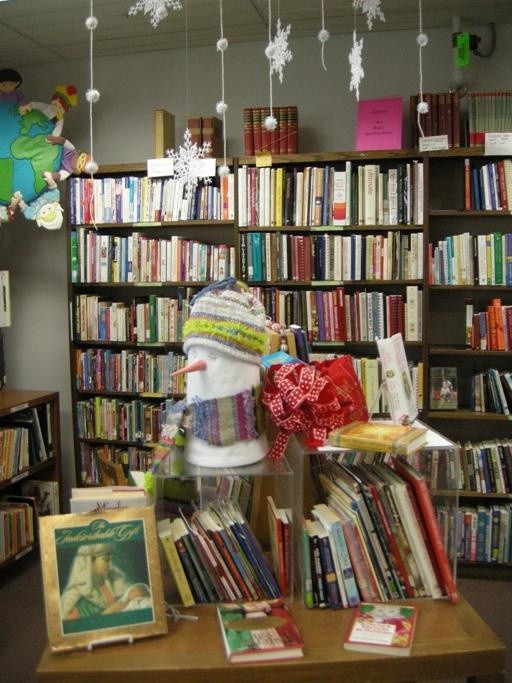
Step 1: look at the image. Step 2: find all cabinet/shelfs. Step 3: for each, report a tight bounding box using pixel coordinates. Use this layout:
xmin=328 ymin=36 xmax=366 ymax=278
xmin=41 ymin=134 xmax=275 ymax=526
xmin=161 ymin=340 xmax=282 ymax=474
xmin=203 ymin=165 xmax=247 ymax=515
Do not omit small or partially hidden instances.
xmin=423 ymin=143 xmax=511 ymax=581
xmin=235 ymin=146 xmax=427 ymax=431
xmin=0 ymin=385 xmax=64 ymax=587
xmin=62 ymin=155 xmax=235 ymax=488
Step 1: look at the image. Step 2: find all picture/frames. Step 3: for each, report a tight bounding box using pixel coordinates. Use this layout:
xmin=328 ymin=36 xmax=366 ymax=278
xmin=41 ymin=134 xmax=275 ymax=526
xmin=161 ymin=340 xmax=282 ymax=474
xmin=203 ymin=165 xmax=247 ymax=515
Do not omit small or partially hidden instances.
xmin=38 ymin=504 xmax=170 ymax=651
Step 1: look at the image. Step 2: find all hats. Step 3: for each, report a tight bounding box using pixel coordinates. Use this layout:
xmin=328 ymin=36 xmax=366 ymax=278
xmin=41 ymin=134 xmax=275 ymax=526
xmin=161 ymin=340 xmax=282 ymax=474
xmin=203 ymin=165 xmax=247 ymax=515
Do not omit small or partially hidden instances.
xmin=182 ymin=276 xmax=265 ymax=364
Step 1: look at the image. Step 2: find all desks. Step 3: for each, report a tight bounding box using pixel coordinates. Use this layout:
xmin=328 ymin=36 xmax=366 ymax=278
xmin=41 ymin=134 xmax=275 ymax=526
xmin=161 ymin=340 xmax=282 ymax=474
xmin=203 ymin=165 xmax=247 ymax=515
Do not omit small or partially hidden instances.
xmin=33 ymin=594 xmax=507 ymax=681
